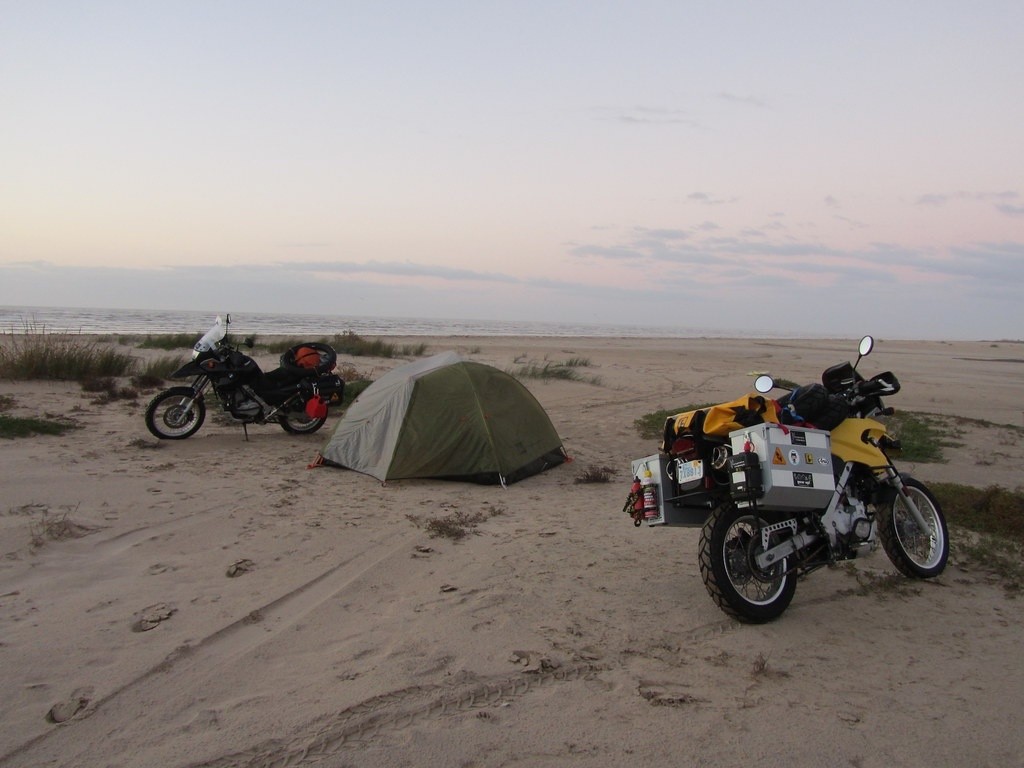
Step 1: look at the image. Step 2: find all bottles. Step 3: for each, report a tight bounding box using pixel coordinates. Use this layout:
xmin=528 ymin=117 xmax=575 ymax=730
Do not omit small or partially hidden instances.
xmin=631 ymin=476 xmax=644 ymax=510
xmin=642 ymin=471 xmax=657 ymax=518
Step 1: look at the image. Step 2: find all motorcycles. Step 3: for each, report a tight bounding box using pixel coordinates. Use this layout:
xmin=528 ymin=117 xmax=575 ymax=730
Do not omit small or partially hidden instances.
xmin=697 ymin=335 xmax=950 ymax=625
xmin=144 ymin=314 xmax=345 ymax=441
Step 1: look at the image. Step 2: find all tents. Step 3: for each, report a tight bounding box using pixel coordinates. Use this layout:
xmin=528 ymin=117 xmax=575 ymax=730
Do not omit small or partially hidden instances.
xmin=322 ymin=349 xmax=566 ymax=481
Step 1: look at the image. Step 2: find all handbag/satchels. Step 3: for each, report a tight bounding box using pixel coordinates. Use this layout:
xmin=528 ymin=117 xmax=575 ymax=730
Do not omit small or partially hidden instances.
xmin=659 ymin=393 xmax=783 ymax=453
